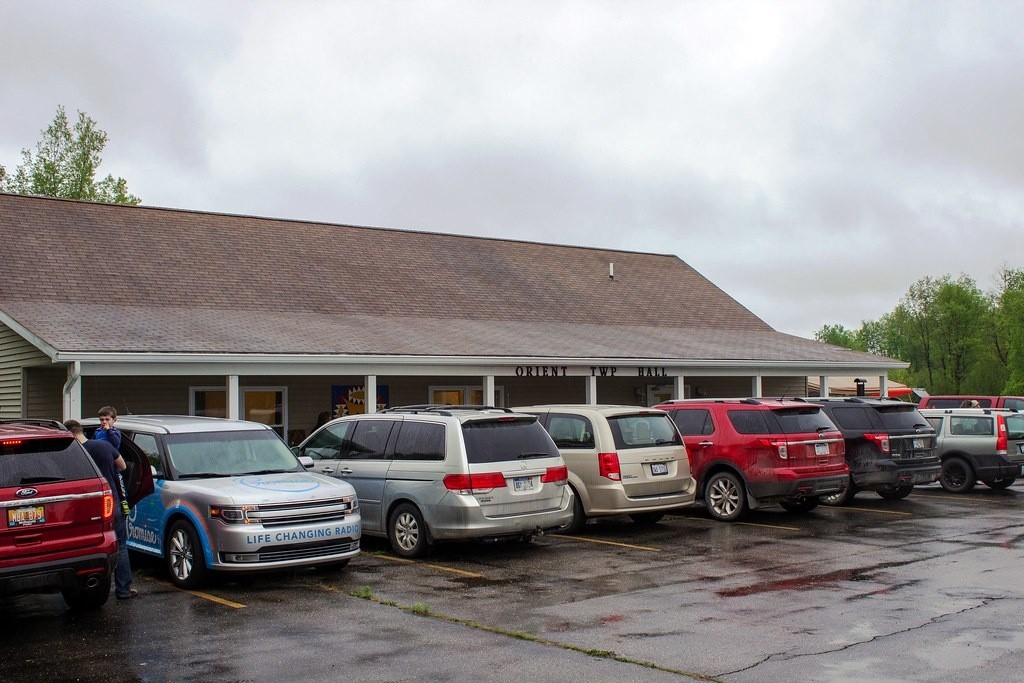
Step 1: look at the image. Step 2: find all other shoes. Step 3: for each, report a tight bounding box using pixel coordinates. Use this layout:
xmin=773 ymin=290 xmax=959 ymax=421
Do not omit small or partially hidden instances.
xmin=131 ymin=588 xmax=137 ymax=597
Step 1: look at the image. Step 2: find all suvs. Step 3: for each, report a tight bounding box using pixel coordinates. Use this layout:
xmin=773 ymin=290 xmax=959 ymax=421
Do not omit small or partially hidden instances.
xmin=651 ymin=397 xmax=851 ymax=522
xmin=295 ymin=404 xmax=575 ymax=557
xmin=915 ymin=395 xmax=1024 ymax=494
xmin=0 ymin=417 xmax=119 ymax=613
xmin=777 ymin=397 xmax=943 ymax=507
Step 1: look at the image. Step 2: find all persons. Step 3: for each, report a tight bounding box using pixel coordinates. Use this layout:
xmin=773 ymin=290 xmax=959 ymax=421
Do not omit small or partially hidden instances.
xmin=1010 ymin=408 xmax=1018 ymax=413
xmin=980 ymin=410 xmax=991 ymax=431
xmin=64 ymin=419 xmax=137 ymax=599
xmin=310 ymin=412 xmax=332 ymax=435
xmin=960 ymin=400 xmax=989 ymax=408
xmin=91 ymin=406 xmax=131 ymax=515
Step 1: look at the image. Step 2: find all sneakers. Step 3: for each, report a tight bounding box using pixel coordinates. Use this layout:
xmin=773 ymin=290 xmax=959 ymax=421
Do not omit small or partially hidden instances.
xmin=122 ymin=501 xmax=130 ymax=515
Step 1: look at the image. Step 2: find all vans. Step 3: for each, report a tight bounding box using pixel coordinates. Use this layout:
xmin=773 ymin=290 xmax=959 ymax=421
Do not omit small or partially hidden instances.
xmin=77 ymin=415 xmax=363 ymax=588
xmin=484 ymin=404 xmax=698 ymax=535
